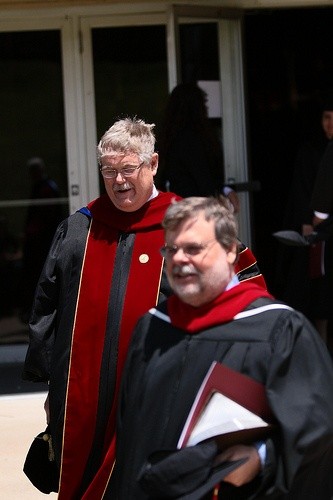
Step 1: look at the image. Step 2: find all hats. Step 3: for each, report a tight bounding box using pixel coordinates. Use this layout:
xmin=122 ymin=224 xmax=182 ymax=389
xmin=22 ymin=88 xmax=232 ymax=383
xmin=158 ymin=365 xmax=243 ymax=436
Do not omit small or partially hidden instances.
xmin=22 ymin=422 xmax=63 ymax=495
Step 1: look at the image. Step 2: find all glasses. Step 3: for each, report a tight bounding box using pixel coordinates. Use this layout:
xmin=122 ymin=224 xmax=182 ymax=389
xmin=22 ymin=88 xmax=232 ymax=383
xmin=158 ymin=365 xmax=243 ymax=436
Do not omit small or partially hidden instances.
xmin=99 ymin=157 xmax=144 ymax=179
xmin=160 ymin=238 xmax=218 ymax=260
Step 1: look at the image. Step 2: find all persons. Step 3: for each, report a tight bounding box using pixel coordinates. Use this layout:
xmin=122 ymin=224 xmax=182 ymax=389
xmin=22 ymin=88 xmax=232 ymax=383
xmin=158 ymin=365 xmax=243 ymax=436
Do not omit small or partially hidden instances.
xmin=21 ymin=116 xmax=267 ymax=500
xmin=20 ymin=158 xmax=65 ymax=323
xmin=115 ymin=196 xmax=333 ymax=500
xmin=161 ymin=85 xmax=225 ymax=197
xmin=300 ymin=102 xmax=333 ymax=343
xmin=0 ymin=216 xmax=23 ymax=303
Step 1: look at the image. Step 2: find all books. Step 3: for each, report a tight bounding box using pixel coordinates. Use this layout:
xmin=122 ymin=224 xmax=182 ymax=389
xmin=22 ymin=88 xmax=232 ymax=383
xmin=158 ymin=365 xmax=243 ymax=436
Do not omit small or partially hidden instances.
xmin=177 ymin=362 xmax=266 ymax=449
